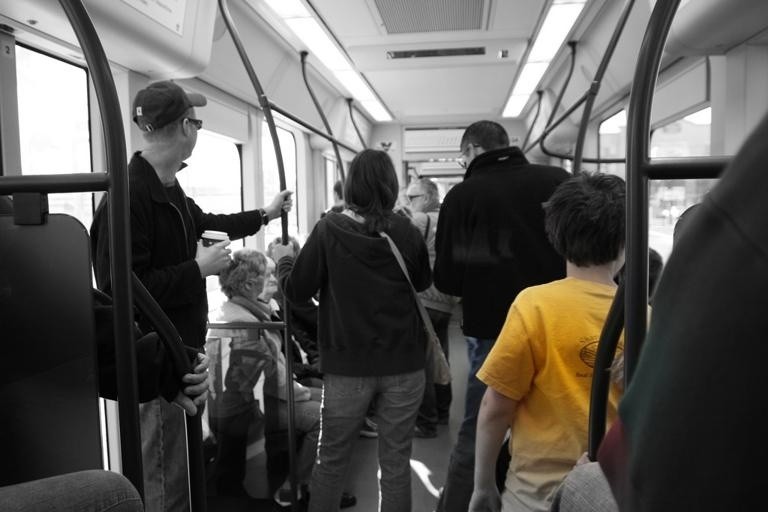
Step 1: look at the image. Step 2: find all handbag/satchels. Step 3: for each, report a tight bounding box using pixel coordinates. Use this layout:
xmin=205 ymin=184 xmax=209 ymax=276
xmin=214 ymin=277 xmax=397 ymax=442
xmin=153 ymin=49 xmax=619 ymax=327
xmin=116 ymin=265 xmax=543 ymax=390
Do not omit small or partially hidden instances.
xmin=424 ymin=332 xmax=453 ymax=386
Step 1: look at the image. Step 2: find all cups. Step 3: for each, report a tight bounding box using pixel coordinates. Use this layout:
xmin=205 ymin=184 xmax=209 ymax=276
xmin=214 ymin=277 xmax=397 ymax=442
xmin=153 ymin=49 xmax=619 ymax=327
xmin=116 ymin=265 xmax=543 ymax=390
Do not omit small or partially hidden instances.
xmin=201 ymin=230 xmax=231 ymax=249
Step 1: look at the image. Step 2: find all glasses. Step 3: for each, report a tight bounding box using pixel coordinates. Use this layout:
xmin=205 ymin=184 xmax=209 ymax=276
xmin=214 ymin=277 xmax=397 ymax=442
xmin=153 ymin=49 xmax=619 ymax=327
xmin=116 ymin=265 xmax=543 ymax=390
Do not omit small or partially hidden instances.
xmin=180 ymin=116 xmax=203 ymax=129
xmin=404 ymin=194 xmax=426 ymax=203
xmin=457 ymin=143 xmax=482 ymax=168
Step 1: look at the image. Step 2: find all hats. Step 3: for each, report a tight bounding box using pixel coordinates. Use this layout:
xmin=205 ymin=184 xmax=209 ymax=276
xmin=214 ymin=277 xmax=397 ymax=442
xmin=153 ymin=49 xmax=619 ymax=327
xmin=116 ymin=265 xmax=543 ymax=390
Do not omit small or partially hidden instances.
xmin=130 ymin=79 xmax=207 ymax=132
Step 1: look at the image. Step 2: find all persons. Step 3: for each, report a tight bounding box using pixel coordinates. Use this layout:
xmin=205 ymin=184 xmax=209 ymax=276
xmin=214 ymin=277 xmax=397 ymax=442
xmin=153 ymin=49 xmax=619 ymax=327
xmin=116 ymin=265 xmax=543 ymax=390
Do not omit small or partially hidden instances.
xmin=615 ymin=111 xmax=768 ymax=512
xmin=93 ymin=299 xmax=210 ymax=416
xmin=0 ymin=469 xmax=144 ymax=512
xmin=90 ymin=82 xmax=294 ymax=512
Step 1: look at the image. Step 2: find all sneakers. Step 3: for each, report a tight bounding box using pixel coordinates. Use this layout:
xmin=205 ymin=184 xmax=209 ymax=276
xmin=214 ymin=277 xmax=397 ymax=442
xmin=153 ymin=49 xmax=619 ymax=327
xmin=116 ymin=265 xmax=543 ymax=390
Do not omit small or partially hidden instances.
xmin=413 ymin=424 xmax=438 ymax=438
xmin=360 ymin=417 xmax=380 ymax=438
xmin=299 ymin=484 xmax=356 ymax=509
